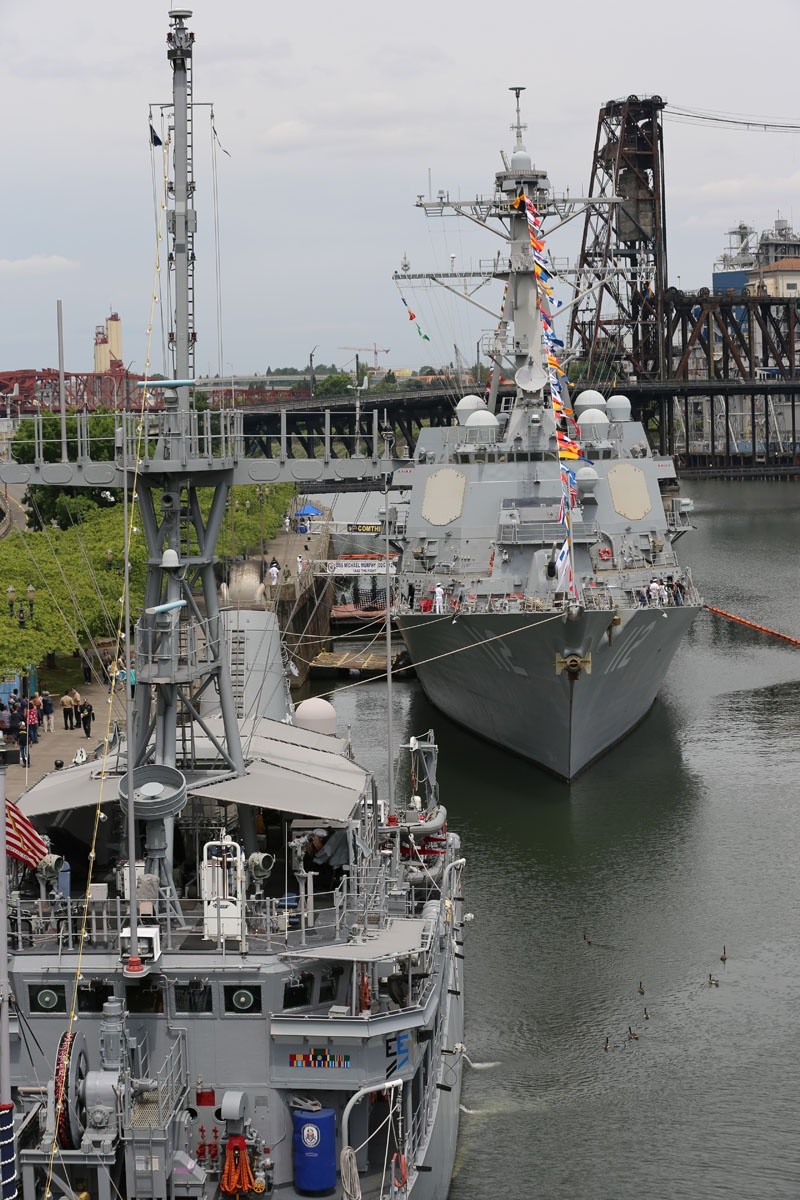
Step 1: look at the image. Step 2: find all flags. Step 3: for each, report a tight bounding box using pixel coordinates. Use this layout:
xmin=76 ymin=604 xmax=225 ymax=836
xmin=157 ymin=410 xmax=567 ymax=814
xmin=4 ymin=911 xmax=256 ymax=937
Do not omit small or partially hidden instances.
xmin=613 ymin=376 xmax=616 ymax=388
xmin=6 ymin=798 xmax=48 ymax=869
xmin=484 ymin=186 xmax=594 ymax=608
xmin=402 ymin=297 xmax=430 ymax=342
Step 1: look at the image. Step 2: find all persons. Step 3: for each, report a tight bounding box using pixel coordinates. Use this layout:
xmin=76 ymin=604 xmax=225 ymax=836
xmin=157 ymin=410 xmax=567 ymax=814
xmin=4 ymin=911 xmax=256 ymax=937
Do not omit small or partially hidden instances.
xmin=408 ymin=579 xmax=475 ymax=614
xmin=285 ymin=517 xmax=311 ymax=533
xmin=0 ymin=688 xmax=95 ymax=768
xmin=268 ymin=554 xmax=302 ymax=584
xmin=581 ymin=577 xmax=597 ymax=588
xmin=640 ymin=579 xmax=686 ymax=606
xmin=80 ymin=648 xmax=135 ymax=699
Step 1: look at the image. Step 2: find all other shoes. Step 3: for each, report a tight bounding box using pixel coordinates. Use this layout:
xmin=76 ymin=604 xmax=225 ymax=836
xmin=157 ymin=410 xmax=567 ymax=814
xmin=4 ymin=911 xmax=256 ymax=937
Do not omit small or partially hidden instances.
xmin=70 ymin=728 xmax=74 ymax=730
xmin=38 ymin=723 xmax=41 ymax=726
xmin=32 ymin=740 xmax=39 ymax=744
xmin=50 ymin=729 xmax=54 ymax=733
xmin=23 ymin=764 xmax=30 ymax=768
xmin=65 ymin=727 xmax=68 ymax=730
xmin=37 ymin=734 xmax=41 ymax=737
xmin=87 ymin=737 xmax=90 ymax=739
xmin=41 ymin=721 xmax=43 ymax=724
xmin=74 ymin=725 xmax=81 ymax=728
xmin=44 ymin=729 xmax=47 ymax=732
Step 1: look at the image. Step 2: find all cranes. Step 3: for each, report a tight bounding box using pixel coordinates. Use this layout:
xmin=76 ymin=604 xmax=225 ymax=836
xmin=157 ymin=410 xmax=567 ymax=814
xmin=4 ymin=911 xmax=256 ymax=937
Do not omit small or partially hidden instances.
xmin=339 ymin=343 xmax=390 ymax=370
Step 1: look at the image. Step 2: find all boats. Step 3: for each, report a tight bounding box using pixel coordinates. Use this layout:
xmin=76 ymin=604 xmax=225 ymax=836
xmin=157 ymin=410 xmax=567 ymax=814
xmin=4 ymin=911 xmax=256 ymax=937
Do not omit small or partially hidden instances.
xmin=381 ymin=84 xmax=703 ymax=782
xmin=3 ymin=11 xmax=474 ymax=1198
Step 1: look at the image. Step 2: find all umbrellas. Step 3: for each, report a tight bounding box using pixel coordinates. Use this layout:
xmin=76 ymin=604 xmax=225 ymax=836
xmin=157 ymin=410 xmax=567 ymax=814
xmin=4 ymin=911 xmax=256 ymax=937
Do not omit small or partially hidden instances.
xmin=295 ymin=503 xmax=323 ymax=516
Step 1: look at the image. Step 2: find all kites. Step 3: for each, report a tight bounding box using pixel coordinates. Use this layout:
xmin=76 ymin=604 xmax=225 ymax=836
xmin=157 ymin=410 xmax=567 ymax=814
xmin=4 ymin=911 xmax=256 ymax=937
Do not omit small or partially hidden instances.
xmin=645 ymin=280 xmax=652 ymax=298
